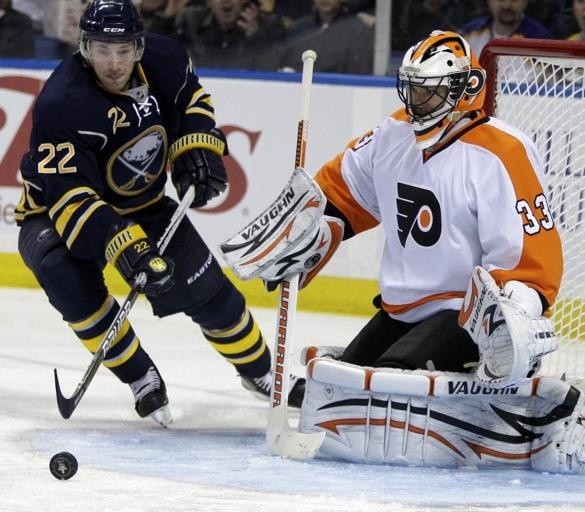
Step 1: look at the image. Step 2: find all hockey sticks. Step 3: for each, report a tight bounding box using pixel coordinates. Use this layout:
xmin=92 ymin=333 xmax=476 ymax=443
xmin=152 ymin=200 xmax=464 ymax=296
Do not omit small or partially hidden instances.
xmin=54 ymin=185 xmax=195 ymax=420
xmin=267 ymin=50 xmax=327 ymax=461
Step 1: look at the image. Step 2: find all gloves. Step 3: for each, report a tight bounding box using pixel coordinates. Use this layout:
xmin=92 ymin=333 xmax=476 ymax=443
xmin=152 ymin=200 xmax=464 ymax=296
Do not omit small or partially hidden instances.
xmin=115 ymin=238 xmax=178 ymax=298
xmin=171 ymin=148 xmax=229 ymax=209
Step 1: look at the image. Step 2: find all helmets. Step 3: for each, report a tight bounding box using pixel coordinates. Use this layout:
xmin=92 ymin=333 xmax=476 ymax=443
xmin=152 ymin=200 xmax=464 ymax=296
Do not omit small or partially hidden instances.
xmin=79 ymin=0 xmax=145 ymax=62
xmin=396 ymin=30 xmax=487 ymax=150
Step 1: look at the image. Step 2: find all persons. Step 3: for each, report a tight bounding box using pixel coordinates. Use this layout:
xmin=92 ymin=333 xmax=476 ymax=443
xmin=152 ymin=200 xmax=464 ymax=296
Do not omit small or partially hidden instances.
xmin=14 ymin=0 xmax=306 ymax=419
xmin=0 ymin=0 xmax=585 ymax=85
xmin=258 ymin=30 xmax=564 ymax=374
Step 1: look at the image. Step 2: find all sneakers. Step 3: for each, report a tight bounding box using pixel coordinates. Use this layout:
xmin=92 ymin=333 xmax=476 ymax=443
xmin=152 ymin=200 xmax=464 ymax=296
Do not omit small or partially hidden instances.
xmin=128 ymin=363 xmax=168 ymax=418
xmin=241 ymin=361 xmax=306 ymax=408
